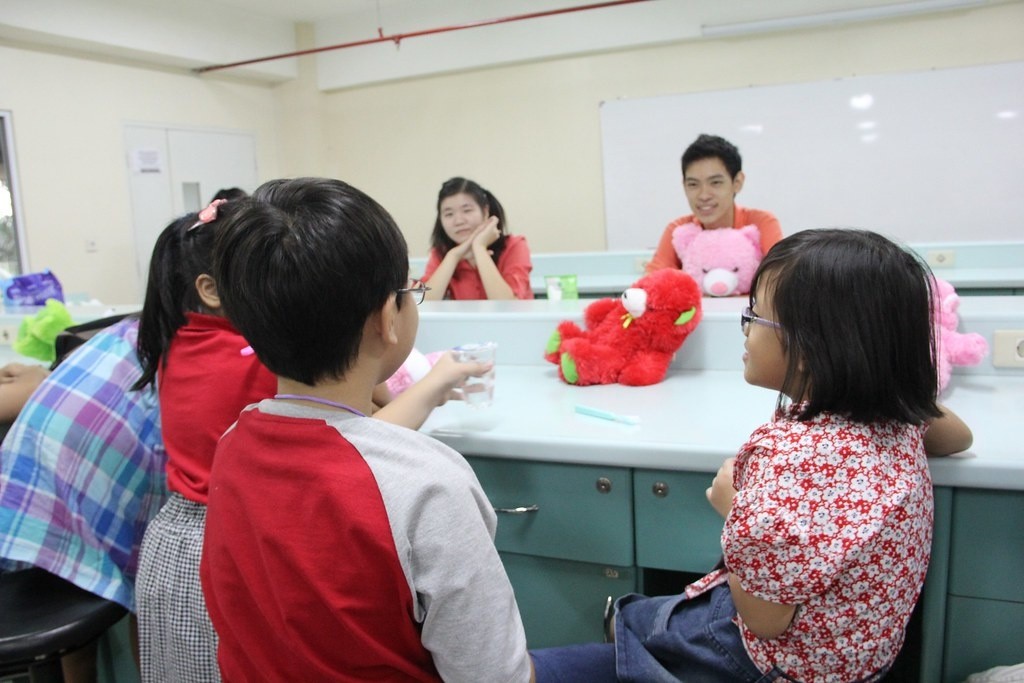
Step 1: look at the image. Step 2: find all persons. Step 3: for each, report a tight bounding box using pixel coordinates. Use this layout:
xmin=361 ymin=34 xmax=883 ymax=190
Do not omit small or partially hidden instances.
xmin=527 ymin=227 xmax=973 ymax=683
xmin=644 ymin=133 xmax=783 ymax=297
xmin=0 ymin=178 xmax=535 ymax=683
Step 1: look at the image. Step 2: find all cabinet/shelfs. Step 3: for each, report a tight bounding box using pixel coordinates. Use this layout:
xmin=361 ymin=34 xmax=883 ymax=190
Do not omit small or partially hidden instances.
xmin=465 ymin=457 xmax=1024 ymax=683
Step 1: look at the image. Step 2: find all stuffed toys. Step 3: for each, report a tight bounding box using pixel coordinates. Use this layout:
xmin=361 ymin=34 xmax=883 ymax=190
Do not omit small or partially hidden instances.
xmin=542 ymin=268 xmax=702 ymax=387
xmin=385 ymin=347 xmax=445 ymax=396
xmin=670 ymin=222 xmax=765 ymax=298
xmin=925 ymin=277 xmax=990 ymax=396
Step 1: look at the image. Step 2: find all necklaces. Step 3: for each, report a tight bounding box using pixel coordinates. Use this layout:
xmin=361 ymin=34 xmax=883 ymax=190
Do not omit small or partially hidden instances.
xmin=274 ymin=394 xmax=369 ymax=417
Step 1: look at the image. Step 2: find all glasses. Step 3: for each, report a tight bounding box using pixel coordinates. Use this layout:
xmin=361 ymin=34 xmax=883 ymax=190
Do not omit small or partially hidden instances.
xmin=741 ymin=305 xmax=782 ymax=337
xmin=397 ymin=278 xmax=432 ymax=305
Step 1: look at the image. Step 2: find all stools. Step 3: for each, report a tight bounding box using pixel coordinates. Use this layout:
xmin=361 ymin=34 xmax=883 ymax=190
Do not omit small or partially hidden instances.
xmin=0 ymin=567 xmax=130 ymax=683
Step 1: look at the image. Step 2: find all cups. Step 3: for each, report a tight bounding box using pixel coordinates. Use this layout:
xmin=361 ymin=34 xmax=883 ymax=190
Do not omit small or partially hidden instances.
xmin=453 ymin=343 xmax=497 ymax=406
